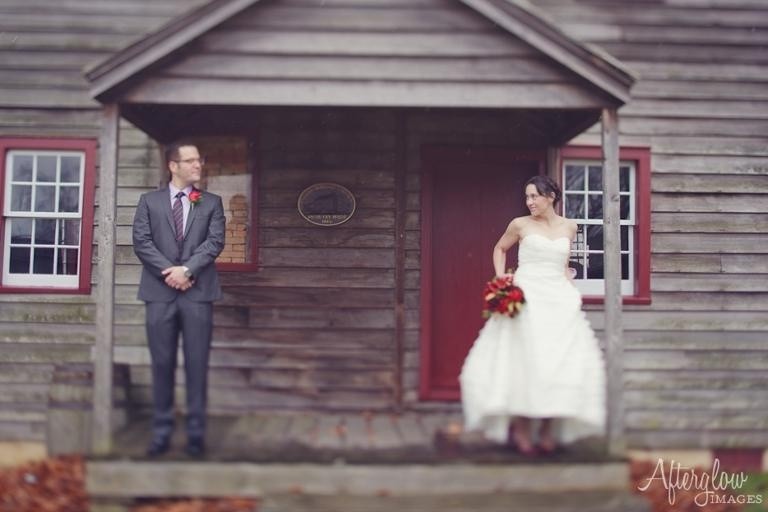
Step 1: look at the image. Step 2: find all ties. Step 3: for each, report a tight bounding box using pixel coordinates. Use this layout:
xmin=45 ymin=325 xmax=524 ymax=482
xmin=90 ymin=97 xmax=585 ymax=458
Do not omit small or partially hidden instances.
xmin=173 ymin=192 xmax=185 ymax=242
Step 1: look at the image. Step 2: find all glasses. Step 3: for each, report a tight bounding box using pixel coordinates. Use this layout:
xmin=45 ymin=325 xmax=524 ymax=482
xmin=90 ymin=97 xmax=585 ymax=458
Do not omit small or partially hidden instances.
xmin=175 ymin=157 xmax=205 ymax=166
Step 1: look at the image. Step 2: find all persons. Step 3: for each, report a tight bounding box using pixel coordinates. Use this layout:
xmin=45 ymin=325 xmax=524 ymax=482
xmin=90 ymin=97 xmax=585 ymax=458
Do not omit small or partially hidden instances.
xmin=457 ymin=175 xmax=608 ymax=457
xmin=132 ymin=139 xmax=226 ymax=461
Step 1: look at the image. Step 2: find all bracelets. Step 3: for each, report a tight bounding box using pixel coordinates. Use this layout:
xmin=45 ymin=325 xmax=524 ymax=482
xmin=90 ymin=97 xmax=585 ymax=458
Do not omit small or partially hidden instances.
xmin=182 ymin=266 xmax=195 ymax=284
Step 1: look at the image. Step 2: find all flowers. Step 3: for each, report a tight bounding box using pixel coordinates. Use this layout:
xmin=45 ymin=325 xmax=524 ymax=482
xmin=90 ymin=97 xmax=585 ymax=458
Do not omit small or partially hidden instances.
xmin=479 ymin=270 xmax=528 ymax=320
xmin=187 ymin=188 xmax=201 ymax=210
